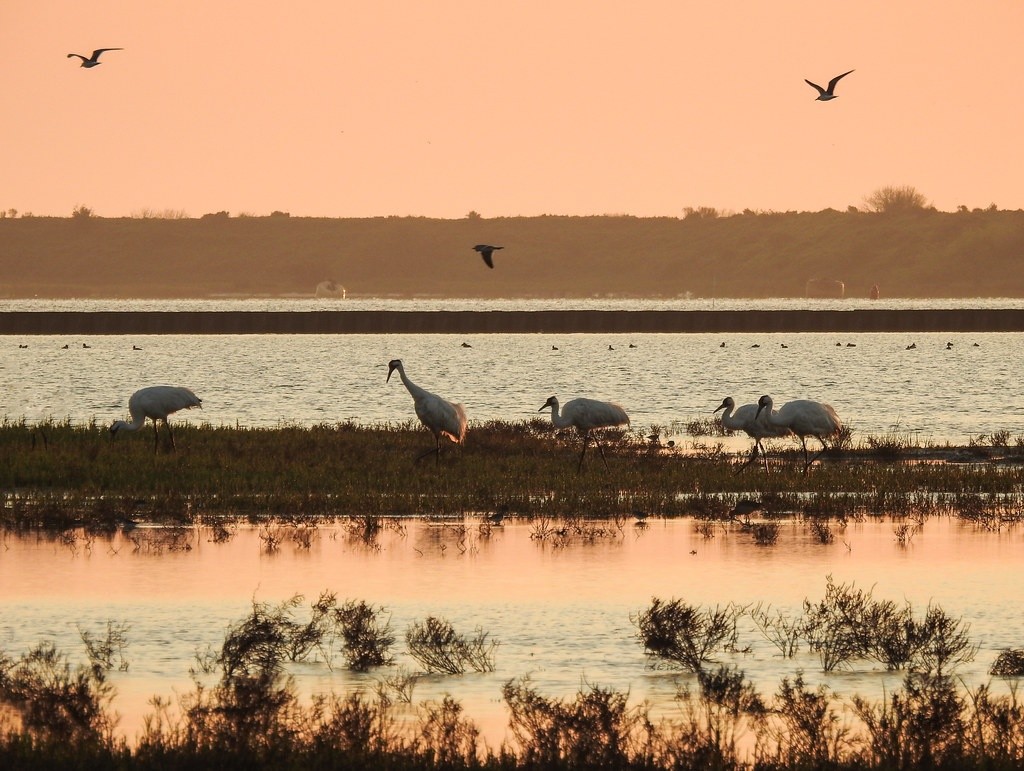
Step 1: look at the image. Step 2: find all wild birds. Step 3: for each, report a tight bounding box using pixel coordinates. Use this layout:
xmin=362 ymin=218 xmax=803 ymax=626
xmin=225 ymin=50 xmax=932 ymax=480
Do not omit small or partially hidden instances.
xmin=67 ymin=48 xmax=123 ymax=68
xmin=755 ymin=394 xmax=842 ymax=480
xmin=805 ymin=70 xmax=855 ymax=101
xmin=110 ymin=386 xmax=202 ymax=457
xmin=713 ymin=396 xmax=791 ymax=478
xmin=538 ymin=396 xmax=630 ymax=477
xmin=752 ymin=344 xmax=760 ymax=347
xmin=946 ymin=342 xmax=979 ymax=349
xmin=906 ymin=343 xmax=916 ymax=350
xmin=720 ymin=342 xmax=725 ymax=347
xmin=461 ymin=343 xmax=471 ymax=347
xmin=552 ymin=345 xmax=558 ymax=350
xmin=608 ymin=344 xmax=635 ymax=350
xmin=387 ymin=358 xmax=467 ymax=469
xmin=62 ymin=343 xmax=91 ymax=349
xmin=781 ymin=344 xmax=788 ymax=348
xmin=836 ymin=343 xmax=855 ymax=347
xmin=133 ymin=346 xmax=142 ymax=350
xmin=19 ymin=345 xmax=27 ymax=348
xmin=472 ymin=244 xmax=504 ymax=269
